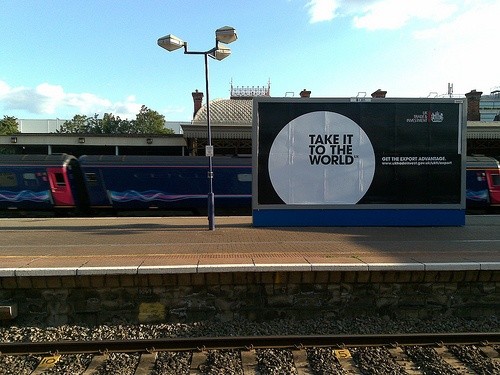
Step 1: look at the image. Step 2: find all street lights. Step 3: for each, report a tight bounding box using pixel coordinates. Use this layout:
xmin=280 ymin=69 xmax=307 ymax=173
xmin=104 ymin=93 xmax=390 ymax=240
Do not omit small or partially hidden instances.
xmin=158 ymin=25 xmax=237 ymax=234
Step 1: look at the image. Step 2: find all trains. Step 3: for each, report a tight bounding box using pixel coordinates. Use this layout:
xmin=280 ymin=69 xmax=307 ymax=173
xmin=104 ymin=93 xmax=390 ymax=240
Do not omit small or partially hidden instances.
xmin=0 ymin=149 xmax=500 ymax=213
